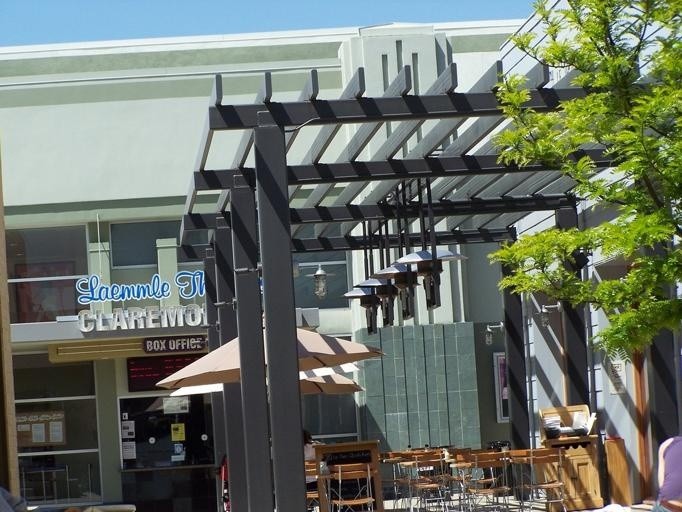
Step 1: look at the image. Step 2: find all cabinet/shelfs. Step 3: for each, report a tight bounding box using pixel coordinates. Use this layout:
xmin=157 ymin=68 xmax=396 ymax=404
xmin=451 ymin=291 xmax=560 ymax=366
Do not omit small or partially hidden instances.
xmin=538 ymin=404 xmax=604 ymax=512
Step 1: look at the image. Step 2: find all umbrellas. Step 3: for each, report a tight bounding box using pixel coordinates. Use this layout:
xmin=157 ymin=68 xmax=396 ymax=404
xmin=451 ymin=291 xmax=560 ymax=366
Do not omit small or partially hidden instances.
xmin=169 ymin=367 xmax=364 ymax=396
xmin=154 ymin=310 xmax=387 ymax=390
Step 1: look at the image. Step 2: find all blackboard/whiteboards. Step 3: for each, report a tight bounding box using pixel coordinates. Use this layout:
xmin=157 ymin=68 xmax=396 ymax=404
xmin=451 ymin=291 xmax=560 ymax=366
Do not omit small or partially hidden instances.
xmin=115 ymin=393 xmax=218 ymax=473
xmin=314 ymin=441 xmax=384 ymax=512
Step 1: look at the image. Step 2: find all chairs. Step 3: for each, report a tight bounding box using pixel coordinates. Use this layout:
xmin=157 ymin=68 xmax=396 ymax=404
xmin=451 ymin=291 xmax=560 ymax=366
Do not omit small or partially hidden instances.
xmin=304 ymin=445 xmax=568 ymax=512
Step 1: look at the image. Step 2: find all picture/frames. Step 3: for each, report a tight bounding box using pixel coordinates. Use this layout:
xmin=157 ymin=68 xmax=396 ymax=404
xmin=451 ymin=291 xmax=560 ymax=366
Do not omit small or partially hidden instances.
xmin=493 ymin=352 xmax=510 ymax=424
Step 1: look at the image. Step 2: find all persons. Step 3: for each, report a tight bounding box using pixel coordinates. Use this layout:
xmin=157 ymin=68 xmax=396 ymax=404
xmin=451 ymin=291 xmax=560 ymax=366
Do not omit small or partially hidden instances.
xmin=654 ymin=436 xmax=681 ymax=512
xmin=303 ymin=427 xmax=325 ymax=491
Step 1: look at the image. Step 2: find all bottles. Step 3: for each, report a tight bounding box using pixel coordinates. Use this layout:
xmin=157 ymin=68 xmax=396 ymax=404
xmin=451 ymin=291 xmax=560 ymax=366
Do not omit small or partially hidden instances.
xmin=487 ymin=440 xmax=512 ymax=453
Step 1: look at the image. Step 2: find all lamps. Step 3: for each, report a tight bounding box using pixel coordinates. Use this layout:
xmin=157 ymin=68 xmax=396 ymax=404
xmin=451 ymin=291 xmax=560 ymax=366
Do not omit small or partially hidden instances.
xmin=485 ymin=322 xmax=505 ymax=346
xmin=314 ymin=264 xmax=328 ymax=300
xmin=535 ymin=301 xmax=563 ymax=328
